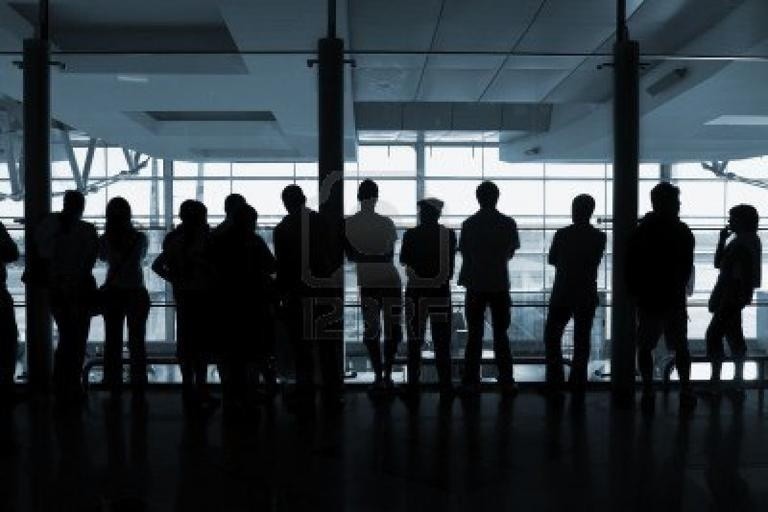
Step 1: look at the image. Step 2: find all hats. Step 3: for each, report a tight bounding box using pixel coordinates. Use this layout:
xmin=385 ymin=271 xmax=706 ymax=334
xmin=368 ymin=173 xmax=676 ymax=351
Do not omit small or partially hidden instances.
xmin=416 ymin=198 xmax=444 ymax=215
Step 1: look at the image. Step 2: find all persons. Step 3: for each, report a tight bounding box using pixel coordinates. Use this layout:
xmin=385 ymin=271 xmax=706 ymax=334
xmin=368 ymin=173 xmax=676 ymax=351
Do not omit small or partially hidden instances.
xmin=398 ymin=195 xmax=456 ymax=401
xmin=344 ymin=180 xmax=404 ymax=394
xmin=542 ymin=194 xmax=606 ymax=400
xmin=153 ymin=183 xmax=321 ymax=411
xmin=0 ymin=222 xmax=22 ymax=396
xmin=624 ymin=183 xmax=696 ymax=402
xmin=697 ymin=203 xmax=761 ymax=397
xmin=30 ymin=189 xmax=101 ymax=394
xmin=96 ymin=194 xmax=152 ymax=406
xmin=459 ymin=180 xmax=521 ymax=394
